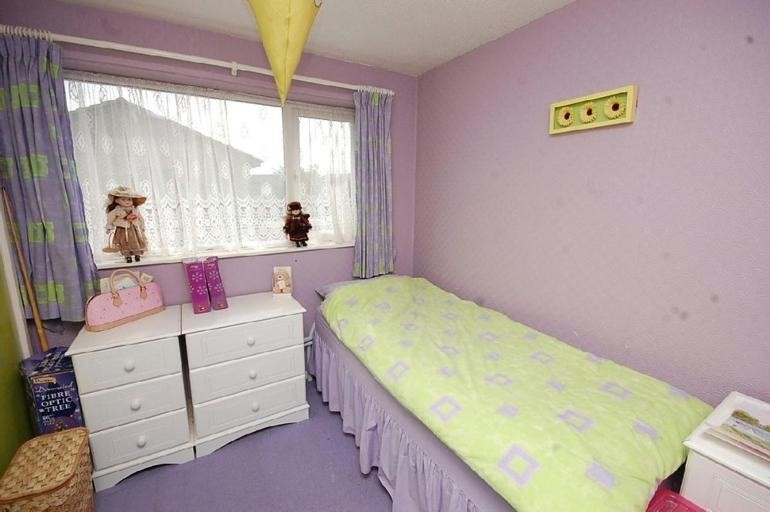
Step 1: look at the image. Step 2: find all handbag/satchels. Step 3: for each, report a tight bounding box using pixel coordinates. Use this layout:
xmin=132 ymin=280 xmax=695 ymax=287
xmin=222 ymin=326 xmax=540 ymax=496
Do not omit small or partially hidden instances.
xmin=84 ymin=268 xmax=166 ymax=332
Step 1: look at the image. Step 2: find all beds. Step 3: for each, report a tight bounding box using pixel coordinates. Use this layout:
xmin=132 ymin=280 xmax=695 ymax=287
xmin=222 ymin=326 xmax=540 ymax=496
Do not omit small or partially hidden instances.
xmin=318 ymin=304 xmax=713 ymax=512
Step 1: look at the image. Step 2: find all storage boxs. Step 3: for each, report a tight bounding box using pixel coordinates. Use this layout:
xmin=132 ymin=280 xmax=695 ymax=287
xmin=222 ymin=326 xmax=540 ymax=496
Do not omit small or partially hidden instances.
xmin=20 ymin=346 xmax=85 ymax=435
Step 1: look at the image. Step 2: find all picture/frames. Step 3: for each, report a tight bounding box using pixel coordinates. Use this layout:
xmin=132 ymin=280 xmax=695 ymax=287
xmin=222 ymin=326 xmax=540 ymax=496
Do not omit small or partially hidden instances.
xmin=549 ymin=84 xmax=638 ymax=134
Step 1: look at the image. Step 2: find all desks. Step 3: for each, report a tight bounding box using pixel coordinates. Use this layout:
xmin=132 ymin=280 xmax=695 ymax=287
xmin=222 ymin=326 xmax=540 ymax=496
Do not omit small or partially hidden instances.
xmin=678 ymin=391 xmax=770 ymax=512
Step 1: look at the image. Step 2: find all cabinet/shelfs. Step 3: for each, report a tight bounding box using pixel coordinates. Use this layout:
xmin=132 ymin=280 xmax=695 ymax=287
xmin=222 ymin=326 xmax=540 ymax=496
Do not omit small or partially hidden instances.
xmin=64 ymin=303 xmax=194 ymax=492
xmin=182 ymin=287 xmax=312 ymax=459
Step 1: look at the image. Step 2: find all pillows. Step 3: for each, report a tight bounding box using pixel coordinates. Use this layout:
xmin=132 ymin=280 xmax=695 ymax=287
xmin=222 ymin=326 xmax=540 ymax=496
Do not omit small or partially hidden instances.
xmin=315 ymin=279 xmax=360 ymax=299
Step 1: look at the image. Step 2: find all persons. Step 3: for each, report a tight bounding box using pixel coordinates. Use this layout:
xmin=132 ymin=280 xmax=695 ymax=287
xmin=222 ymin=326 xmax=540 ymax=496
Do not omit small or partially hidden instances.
xmin=101 ymin=185 xmax=147 ymax=263
xmin=283 ymin=201 xmax=312 ymax=248
